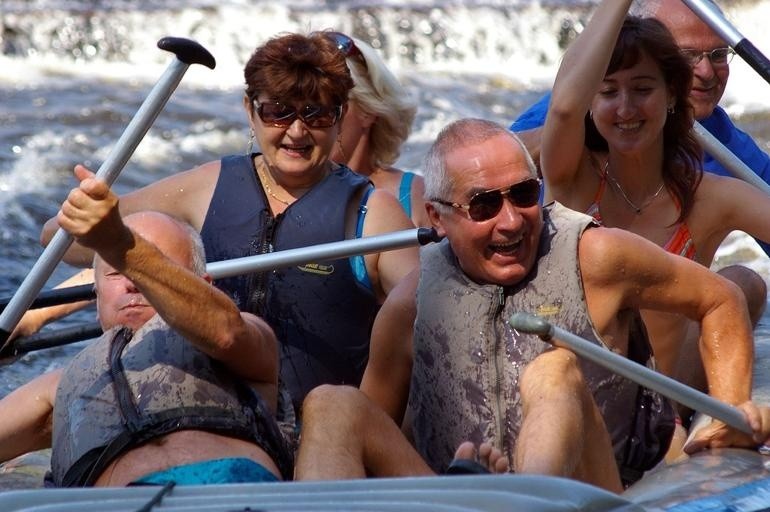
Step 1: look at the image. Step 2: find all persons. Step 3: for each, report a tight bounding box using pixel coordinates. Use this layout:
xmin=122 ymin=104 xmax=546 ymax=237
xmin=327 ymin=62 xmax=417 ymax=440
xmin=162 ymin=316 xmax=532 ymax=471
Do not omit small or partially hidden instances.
xmin=0 ymin=164 xmax=280 ymax=487
xmin=308 ymin=31 xmax=433 ymax=228
xmin=40 ymin=30 xmax=420 ymax=439
xmin=509 ymin=1 xmax=770 ymax=256
xmin=539 ymin=0 xmax=770 ymax=464
xmin=294 ymin=117 xmax=770 ymax=495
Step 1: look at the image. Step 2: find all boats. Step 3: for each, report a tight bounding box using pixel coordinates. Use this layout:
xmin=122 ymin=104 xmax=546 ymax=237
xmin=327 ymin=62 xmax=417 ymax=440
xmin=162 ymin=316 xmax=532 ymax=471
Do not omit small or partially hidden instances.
xmin=0 ymin=300 xmax=770 ymax=512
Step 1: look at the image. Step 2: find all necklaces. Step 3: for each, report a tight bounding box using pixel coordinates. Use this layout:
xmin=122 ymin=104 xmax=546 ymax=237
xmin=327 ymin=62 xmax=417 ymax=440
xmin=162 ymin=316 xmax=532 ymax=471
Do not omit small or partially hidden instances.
xmin=260 ymin=161 xmax=292 ymax=206
xmin=604 ymin=160 xmax=666 ymax=213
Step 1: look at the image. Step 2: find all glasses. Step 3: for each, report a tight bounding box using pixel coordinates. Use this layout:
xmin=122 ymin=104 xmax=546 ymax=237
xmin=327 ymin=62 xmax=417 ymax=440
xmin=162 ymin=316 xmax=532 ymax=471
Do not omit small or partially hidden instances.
xmin=681 ymin=47 xmax=736 ymax=66
xmin=308 ymin=30 xmax=361 ymax=57
xmin=430 ymin=177 xmax=542 ymax=222
xmin=253 ymin=99 xmax=343 ymax=128
